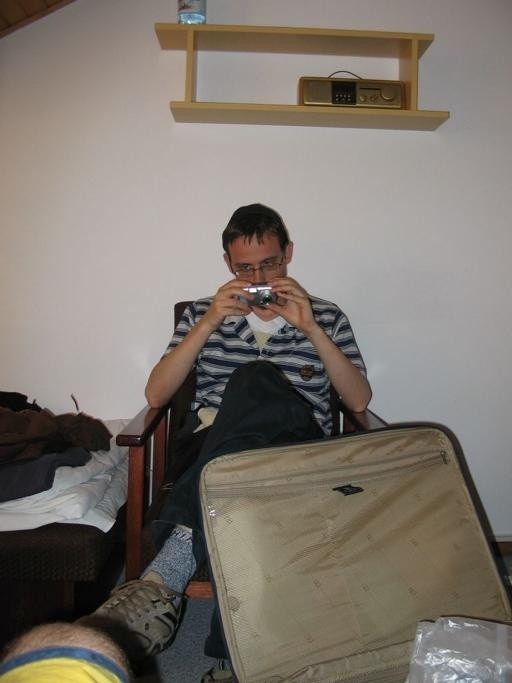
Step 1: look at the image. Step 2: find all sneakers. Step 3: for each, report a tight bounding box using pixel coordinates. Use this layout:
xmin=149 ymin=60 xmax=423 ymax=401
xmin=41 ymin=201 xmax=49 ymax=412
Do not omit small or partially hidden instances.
xmin=74 ymin=579 xmax=185 ymax=662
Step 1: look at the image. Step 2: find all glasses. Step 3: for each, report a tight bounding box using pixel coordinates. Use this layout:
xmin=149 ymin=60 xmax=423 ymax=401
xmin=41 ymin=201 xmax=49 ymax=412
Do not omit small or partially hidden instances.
xmin=229 ymin=250 xmax=283 ymax=278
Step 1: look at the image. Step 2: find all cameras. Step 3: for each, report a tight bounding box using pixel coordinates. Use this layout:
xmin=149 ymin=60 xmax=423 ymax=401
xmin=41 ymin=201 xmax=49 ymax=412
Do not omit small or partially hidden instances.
xmin=241 ymin=286 xmax=282 ymax=307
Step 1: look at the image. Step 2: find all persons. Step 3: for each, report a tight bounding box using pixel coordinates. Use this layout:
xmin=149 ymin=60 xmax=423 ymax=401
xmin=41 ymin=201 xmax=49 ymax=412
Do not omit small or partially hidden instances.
xmin=0 ymin=620 xmax=137 ymax=682
xmin=68 ymin=201 xmax=374 ymax=682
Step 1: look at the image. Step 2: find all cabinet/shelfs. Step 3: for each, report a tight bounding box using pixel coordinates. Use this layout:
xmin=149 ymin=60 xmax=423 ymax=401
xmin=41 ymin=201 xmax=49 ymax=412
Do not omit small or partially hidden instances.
xmin=153 ymin=23 xmax=453 ymax=130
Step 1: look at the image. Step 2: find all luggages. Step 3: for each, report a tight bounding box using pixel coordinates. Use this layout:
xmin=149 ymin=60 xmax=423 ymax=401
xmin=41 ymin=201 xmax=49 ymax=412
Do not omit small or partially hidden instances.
xmin=195 ymin=422 xmax=512 ymax=683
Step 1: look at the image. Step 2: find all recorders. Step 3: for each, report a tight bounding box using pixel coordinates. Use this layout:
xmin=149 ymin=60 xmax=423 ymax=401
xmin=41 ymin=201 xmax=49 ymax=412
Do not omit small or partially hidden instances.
xmin=299 ymin=76 xmax=409 ymax=108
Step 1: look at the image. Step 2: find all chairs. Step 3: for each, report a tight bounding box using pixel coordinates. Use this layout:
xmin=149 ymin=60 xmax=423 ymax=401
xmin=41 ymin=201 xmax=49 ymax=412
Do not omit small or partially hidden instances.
xmin=118 ymin=302 xmax=393 ymax=594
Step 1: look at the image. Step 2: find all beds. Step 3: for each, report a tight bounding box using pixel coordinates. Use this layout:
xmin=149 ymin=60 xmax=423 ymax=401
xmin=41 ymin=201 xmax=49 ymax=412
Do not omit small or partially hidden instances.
xmin=1 ymin=422 xmax=141 ymax=627
